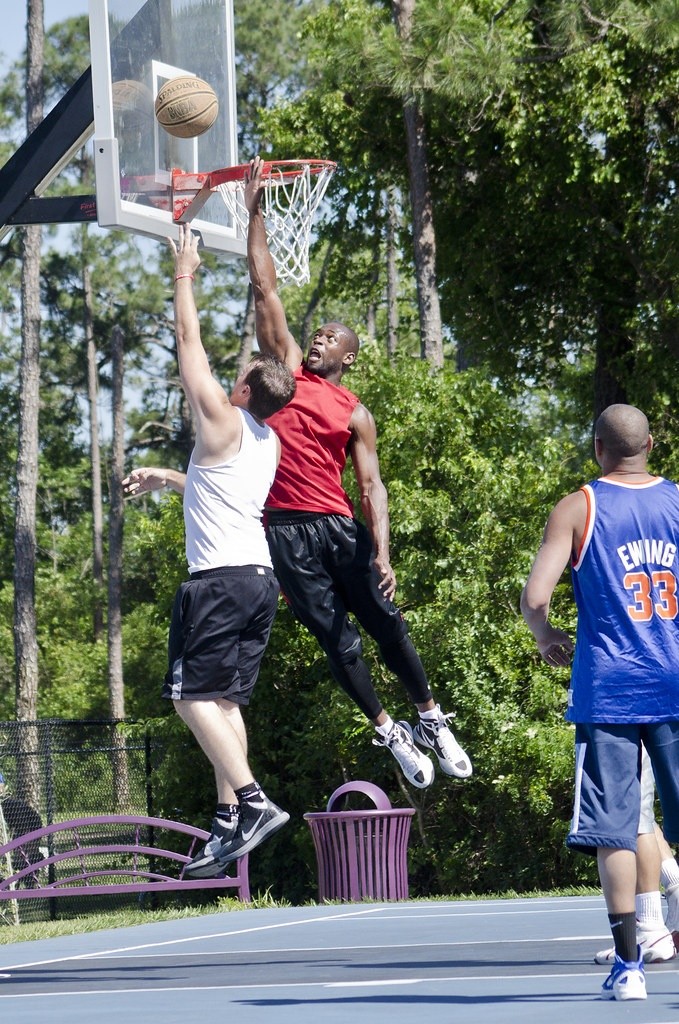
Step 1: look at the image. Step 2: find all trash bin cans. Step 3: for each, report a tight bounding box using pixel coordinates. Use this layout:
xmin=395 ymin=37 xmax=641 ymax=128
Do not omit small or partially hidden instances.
xmin=304 ymin=780 xmax=415 ymax=904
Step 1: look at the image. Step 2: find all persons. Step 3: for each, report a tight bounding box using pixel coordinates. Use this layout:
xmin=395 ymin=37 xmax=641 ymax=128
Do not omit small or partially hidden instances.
xmin=242 ymin=156 xmax=472 ymax=788
xmin=520 ymin=404 xmax=679 ymax=999
xmin=120 ymin=223 xmax=297 ymax=878
xmin=593 ymin=741 xmax=679 ymax=966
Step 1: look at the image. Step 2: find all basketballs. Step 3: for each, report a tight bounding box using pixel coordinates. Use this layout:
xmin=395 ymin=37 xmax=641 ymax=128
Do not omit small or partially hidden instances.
xmin=154 ymin=75 xmax=220 ymax=139
xmin=112 ymin=79 xmax=152 ymax=130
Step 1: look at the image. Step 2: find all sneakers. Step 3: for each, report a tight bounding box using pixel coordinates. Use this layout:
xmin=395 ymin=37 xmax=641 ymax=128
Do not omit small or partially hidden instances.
xmin=413 ymin=705 xmax=473 ymax=778
xmin=593 ymin=922 xmax=677 ymax=964
xmin=183 ymin=816 xmax=242 ymax=877
xmin=218 ymin=791 xmax=290 ymax=862
xmin=602 ymin=944 xmax=646 ymax=1000
xmin=372 ymin=720 xmax=434 ymax=788
xmin=661 ymin=885 xmax=679 ymax=952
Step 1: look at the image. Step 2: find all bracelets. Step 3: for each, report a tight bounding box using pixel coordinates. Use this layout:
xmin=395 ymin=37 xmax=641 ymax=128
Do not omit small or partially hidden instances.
xmin=172 ymin=275 xmax=196 ymax=283
